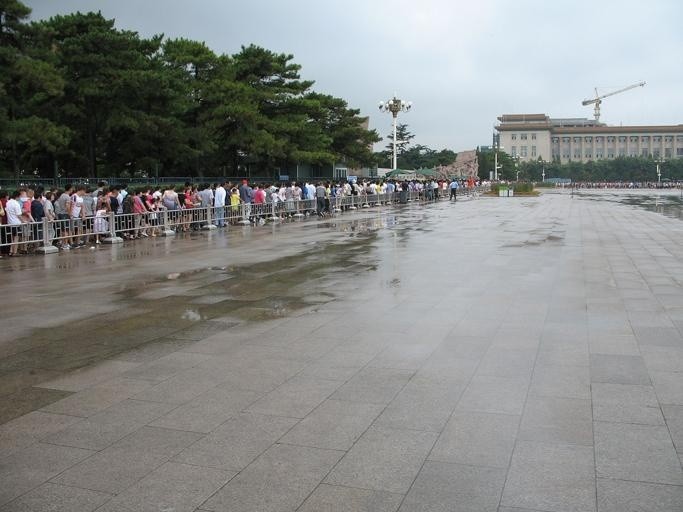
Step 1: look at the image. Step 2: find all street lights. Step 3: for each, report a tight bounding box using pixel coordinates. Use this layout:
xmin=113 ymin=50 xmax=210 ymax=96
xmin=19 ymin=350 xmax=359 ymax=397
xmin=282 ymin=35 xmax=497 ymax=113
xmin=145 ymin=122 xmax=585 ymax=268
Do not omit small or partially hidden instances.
xmin=379 ymin=97 xmax=412 ymax=171
xmin=652 ymin=159 xmax=666 ymax=182
xmin=488 ymin=142 xmax=504 ymax=180
xmin=512 ymin=153 xmax=524 ymax=181
xmin=538 ymin=160 xmax=550 ymax=183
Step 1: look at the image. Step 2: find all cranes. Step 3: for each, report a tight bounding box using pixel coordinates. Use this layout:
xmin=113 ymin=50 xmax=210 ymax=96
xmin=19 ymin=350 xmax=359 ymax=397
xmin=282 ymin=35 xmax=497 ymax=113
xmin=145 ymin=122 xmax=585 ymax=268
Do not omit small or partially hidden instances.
xmin=583 ymin=79 xmax=646 ymax=119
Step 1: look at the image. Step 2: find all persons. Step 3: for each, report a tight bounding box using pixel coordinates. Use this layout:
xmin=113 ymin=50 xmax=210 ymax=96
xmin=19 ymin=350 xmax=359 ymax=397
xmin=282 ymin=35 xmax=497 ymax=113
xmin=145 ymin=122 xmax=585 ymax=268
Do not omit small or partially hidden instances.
xmin=1 ymin=180 xmax=480 ymax=258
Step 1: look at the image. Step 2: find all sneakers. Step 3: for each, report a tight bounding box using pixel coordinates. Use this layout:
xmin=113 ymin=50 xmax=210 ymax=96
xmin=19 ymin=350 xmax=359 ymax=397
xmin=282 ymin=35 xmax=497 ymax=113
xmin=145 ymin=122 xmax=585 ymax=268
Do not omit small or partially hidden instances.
xmin=124 ymin=232 xmax=157 ymax=240
xmin=60 ymin=240 xmax=101 ymax=250
xmin=9 ymin=251 xmax=29 ymax=256
